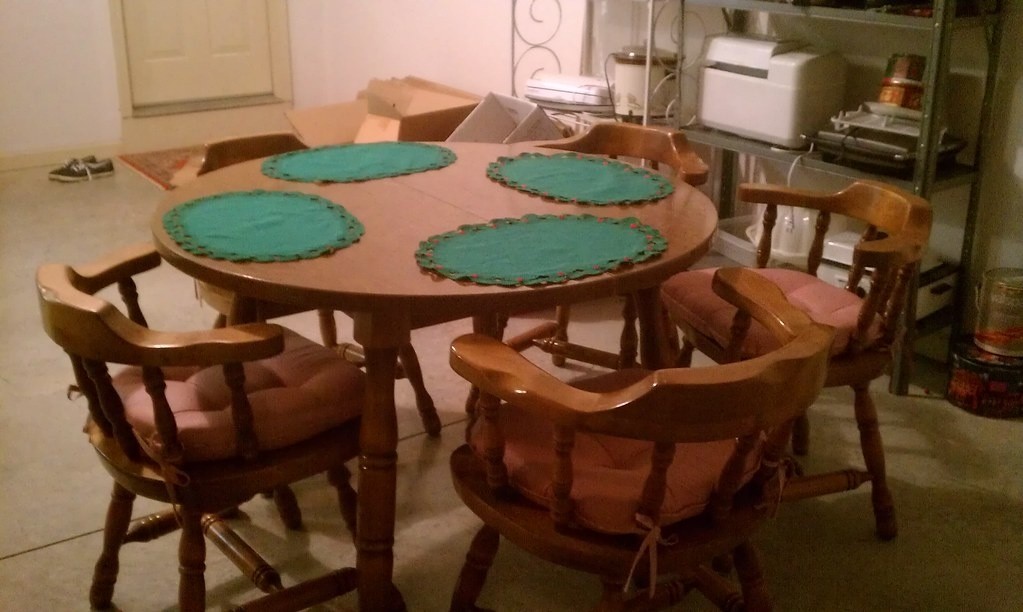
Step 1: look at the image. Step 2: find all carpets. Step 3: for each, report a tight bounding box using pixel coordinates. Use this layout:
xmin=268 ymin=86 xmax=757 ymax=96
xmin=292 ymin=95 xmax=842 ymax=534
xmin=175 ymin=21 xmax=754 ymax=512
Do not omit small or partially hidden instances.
xmin=117 ymin=144 xmax=206 ymax=191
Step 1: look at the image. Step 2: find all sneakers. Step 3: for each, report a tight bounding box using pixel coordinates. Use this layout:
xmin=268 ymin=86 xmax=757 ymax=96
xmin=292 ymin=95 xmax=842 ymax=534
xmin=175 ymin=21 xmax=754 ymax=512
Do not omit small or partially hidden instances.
xmin=49 ymin=156 xmax=95 ymax=180
xmin=57 ymin=158 xmax=113 ymax=182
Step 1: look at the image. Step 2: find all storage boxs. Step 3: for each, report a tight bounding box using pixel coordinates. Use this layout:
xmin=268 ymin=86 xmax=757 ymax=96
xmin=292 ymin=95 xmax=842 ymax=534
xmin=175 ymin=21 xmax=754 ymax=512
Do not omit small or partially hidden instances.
xmin=286 ymin=73 xmax=567 ymax=151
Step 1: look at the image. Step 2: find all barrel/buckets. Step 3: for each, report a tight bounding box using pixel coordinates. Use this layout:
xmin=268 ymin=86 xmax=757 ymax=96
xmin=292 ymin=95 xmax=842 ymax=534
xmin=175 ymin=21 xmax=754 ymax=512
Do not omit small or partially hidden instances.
xmin=974 ymin=267 xmax=1023 ymax=357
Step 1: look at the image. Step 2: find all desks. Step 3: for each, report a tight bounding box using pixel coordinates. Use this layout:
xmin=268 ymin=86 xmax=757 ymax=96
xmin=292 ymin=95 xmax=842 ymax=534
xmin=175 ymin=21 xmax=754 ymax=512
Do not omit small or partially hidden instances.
xmin=150 ymin=140 xmax=717 ymax=611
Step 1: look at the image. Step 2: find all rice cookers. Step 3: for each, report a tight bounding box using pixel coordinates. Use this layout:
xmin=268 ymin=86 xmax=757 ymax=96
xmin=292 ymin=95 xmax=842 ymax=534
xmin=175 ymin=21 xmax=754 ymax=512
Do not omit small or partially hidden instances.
xmin=613 ymin=40 xmax=686 ymax=121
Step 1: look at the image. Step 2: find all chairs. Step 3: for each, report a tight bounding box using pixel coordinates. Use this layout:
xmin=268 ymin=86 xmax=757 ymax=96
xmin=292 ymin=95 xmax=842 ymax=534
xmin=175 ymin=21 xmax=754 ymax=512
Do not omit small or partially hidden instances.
xmin=191 ymin=134 xmax=353 ymax=331
xmin=661 ymin=181 xmax=937 ymax=541
xmin=502 ymin=122 xmax=711 ymax=371
xmin=448 ymin=268 xmax=839 ymax=612
xmin=37 ymin=237 xmax=366 ymax=612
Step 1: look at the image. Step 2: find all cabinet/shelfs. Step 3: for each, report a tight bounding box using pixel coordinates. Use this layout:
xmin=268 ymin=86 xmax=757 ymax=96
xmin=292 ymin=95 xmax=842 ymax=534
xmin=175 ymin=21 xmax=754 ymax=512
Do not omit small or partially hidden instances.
xmin=588 ymin=1 xmax=1008 ymax=396
